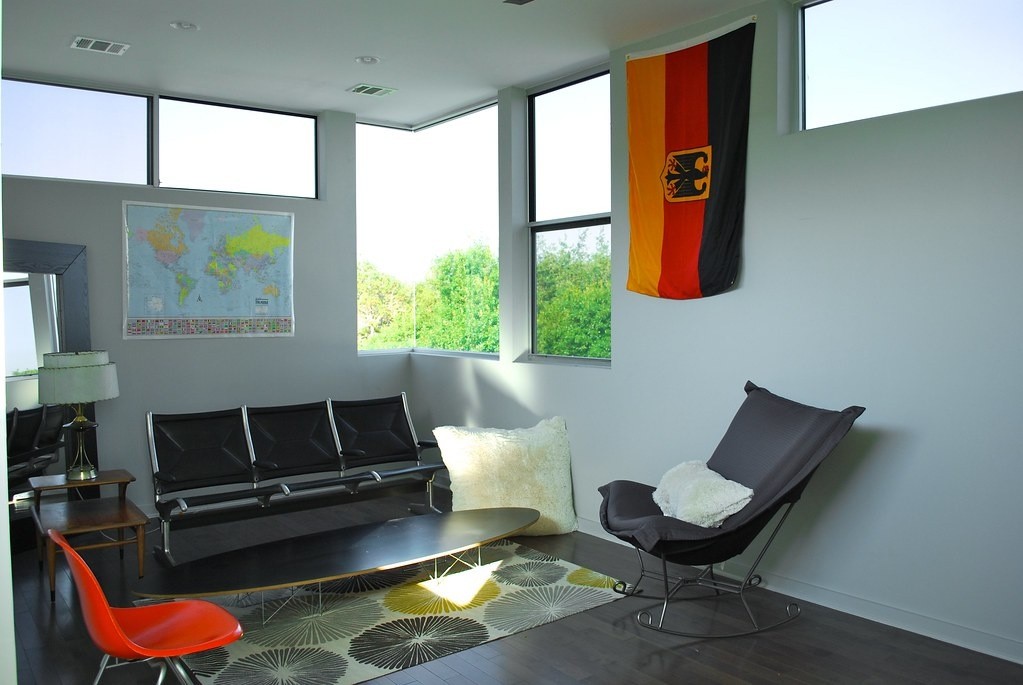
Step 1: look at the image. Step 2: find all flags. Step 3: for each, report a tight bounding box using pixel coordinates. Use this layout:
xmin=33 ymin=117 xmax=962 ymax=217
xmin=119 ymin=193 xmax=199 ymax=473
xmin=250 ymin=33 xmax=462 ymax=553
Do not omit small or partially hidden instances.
xmin=624 ymin=15 xmax=756 ymax=300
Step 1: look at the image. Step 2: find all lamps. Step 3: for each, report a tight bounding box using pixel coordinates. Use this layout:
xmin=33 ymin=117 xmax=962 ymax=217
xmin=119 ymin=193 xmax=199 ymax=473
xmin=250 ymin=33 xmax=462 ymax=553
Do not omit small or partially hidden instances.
xmin=37 ymin=351 xmax=120 ymax=483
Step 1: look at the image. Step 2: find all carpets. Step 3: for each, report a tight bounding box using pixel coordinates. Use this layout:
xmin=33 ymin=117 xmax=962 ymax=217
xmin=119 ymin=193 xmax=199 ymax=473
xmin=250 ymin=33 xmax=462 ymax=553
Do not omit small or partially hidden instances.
xmin=132 ymin=537 xmax=645 ymax=685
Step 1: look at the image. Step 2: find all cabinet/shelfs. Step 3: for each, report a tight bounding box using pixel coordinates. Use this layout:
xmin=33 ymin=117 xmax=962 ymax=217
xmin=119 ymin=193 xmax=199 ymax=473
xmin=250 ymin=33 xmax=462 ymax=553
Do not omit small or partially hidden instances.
xmin=30 ymin=469 xmax=151 ymax=603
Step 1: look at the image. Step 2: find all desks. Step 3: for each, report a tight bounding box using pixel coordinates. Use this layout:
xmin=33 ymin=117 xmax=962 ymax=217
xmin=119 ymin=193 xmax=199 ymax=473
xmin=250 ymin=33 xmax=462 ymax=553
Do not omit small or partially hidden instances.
xmin=130 ymin=506 xmax=540 ymax=630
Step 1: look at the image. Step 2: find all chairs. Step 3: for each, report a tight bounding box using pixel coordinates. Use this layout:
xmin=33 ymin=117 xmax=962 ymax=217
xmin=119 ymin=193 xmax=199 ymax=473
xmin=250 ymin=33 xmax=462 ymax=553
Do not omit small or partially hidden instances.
xmin=48 ymin=526 xmax=244 ymax=685
xmin=597 ymin=380 xmax=866 ymax=640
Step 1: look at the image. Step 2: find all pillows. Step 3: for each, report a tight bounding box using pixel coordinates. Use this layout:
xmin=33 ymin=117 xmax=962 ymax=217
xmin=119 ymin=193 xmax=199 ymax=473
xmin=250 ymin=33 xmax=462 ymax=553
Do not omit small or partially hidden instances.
xmin=651 ymin=460 xmax=755 ymax=528
xmin=433 ymin=413 xmax=579 ymax=538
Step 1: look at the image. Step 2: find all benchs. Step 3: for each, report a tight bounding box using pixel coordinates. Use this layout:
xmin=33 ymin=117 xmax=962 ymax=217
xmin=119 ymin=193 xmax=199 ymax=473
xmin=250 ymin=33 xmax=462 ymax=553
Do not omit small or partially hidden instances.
xmin=6 ymin=401 xmax=68 ymax=488
xmin=142 ymin=391 xmax=450 ymax=571
